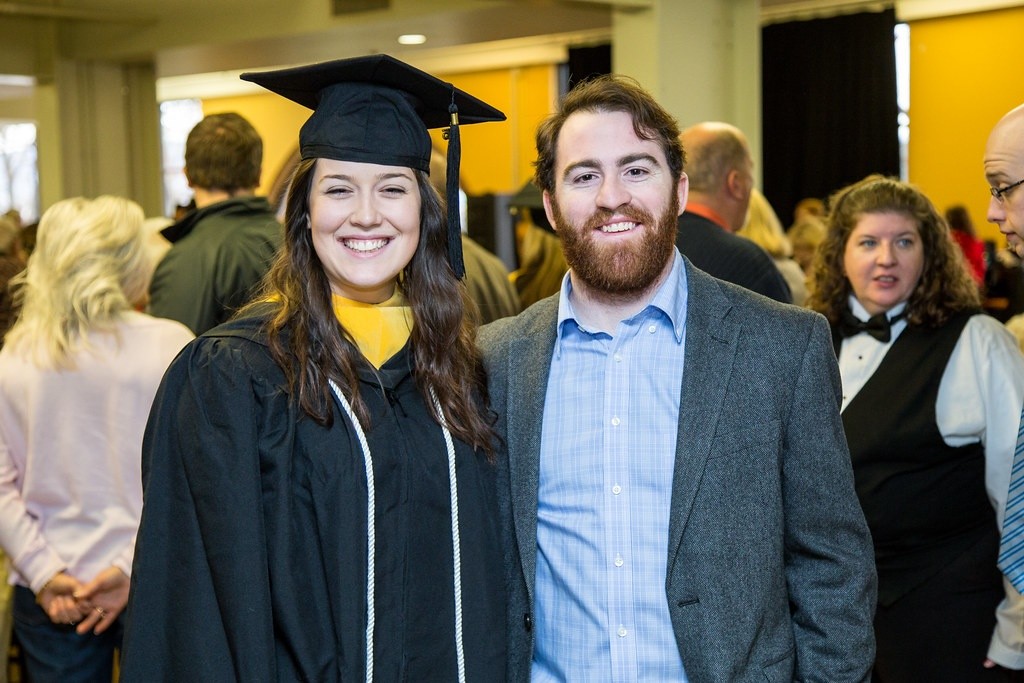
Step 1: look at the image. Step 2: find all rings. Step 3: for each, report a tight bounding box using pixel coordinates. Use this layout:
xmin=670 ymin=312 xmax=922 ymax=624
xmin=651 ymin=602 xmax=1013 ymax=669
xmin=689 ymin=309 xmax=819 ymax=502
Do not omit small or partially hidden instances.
xmin=95 ymin=607 xmax=104 ymax=614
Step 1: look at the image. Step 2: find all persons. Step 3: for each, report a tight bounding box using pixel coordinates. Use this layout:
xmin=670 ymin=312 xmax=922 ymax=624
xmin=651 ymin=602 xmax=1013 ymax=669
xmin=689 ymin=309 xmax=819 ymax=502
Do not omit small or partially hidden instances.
xmin=0 ymin=54 xmax=1024 ymax=683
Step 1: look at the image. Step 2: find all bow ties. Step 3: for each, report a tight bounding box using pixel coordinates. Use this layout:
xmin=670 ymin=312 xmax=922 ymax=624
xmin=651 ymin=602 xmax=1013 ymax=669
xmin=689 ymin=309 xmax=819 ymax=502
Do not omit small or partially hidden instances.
xmin=839 ymin=308 xmax=909 ymax=343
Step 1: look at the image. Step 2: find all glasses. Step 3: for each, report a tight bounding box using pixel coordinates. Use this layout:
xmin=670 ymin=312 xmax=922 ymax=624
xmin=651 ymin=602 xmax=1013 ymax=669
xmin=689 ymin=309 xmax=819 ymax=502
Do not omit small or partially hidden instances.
xmin=989 ymin=179 xmax=1024 ymax=204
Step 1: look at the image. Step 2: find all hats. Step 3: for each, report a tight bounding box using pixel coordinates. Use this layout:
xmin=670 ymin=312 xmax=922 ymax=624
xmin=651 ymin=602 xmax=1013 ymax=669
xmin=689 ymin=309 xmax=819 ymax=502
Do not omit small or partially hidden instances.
xmin=239 ymin=53 xmax=507 ymax=280
xmin=505 ymin=177 xmax=556 ymax=234
